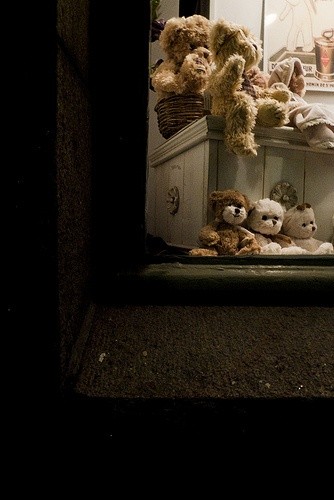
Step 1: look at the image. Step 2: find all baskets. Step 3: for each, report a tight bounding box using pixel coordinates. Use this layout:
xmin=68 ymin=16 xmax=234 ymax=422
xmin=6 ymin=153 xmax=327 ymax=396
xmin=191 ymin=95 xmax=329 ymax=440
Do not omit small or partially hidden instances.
xmin=154 ymin=93 xmax=211 ymax=138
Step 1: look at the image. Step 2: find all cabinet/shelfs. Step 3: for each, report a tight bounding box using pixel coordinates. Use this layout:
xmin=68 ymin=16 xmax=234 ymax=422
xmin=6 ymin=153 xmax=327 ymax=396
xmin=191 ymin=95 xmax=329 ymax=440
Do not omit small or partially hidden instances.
xmin=216 ymin=142 xmax=334 ymax=257
xmin=149 ymin=140 xmax=209 ymax=249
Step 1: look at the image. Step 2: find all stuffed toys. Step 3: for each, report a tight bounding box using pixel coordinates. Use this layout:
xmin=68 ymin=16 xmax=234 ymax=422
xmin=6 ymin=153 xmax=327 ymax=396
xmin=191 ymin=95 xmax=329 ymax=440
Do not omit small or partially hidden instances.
xmin=188 ymin=190 xmax=261 ymax=257
xmin=282 ymin=203 xmax=333 ymax=255
xmin=152 ymin=14 xmax=212 ymax=94
xmin=244 ymin=198 xmax=283 ymax=255
xmin=209 ymin=20 xmax=291 ymax=157
